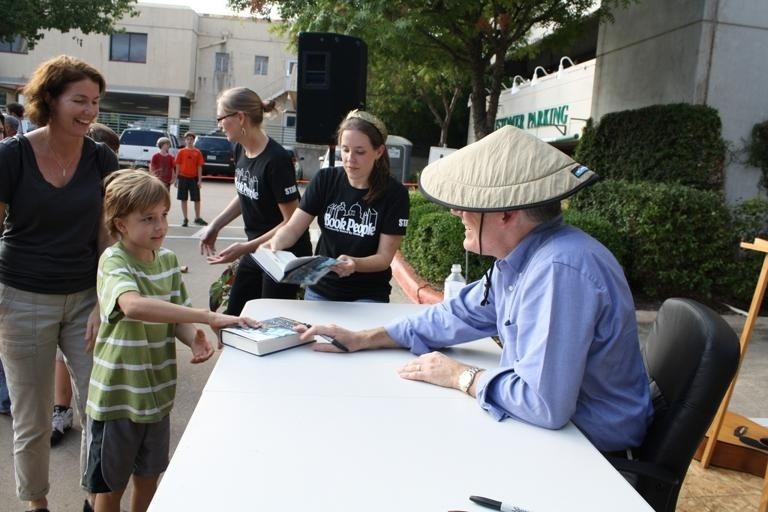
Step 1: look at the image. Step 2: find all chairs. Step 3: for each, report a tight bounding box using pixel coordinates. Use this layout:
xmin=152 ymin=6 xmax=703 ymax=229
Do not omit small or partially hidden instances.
xmin=639 ymin=297 xmax=741 ymax=512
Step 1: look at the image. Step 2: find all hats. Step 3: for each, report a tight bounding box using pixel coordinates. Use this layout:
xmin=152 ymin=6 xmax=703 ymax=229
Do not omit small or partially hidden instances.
xmin=418 ymin=125 xmax=598 ymax=213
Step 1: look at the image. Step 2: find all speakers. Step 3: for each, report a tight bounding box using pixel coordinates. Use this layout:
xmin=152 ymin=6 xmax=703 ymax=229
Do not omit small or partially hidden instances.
xmin=295 ymin=31 xmax=369 ymax=145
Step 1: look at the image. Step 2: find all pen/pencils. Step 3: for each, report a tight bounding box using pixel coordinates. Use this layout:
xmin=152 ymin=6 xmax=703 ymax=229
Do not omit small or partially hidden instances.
xmin=306 ymin=324 xmax=349 ymax=352
xmin=469 ymin=496 xmax=530 ymax=512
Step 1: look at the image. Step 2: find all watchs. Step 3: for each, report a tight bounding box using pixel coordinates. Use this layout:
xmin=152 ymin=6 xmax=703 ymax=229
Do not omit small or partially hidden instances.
xmin=459 ymin=366 xmax=486 ymax=396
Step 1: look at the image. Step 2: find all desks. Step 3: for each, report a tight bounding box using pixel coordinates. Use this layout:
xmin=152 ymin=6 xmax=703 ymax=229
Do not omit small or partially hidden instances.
xmin=144 ymin=298 xmax=658 ymax=512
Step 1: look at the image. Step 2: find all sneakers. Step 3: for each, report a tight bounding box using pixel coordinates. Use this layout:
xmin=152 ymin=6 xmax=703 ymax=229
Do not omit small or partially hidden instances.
xmin=182 ymin=219 xmax=188 ymax=227
xmin=50 ymin=405 xmax=74 ymax=448
xmin=194 ymin=218 xmax=208 ymax=225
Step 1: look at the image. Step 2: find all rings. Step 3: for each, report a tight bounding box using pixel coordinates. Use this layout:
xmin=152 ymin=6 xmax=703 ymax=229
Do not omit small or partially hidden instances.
xmin=416 ymin=365 xmax=421 ymax=371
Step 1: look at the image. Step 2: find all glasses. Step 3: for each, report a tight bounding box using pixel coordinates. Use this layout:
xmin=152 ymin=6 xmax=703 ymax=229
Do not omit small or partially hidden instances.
xmin=216 ymin=111 xmax=237 ymax=123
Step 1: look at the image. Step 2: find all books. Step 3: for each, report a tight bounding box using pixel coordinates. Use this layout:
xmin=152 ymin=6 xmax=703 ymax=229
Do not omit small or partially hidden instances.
xmin=248 ymin=245 xmax=350 ymax=285
xmin=218 ymin=316 xmax=316 ymax=357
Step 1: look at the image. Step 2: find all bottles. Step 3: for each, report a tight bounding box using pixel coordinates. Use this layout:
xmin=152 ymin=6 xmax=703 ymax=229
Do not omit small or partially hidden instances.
xmin=442 ymin=263 xmax=466 ymax=304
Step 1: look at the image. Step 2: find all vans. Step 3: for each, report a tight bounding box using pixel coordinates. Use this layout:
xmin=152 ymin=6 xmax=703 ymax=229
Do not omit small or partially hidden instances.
xmin=192 ymin=134 xmax=241 ymax=177
xmin=115 ymin=127 xmax=185 ymax=172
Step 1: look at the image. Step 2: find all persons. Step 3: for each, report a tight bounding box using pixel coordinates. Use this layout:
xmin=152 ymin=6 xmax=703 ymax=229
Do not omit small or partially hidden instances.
xmin=198 ymin=87 xmax=314 ymax=318
xmin=260 ymin=108 xmax=411 ymax=305
xmin=292 ymin=125 xmax=653 ymax=484
xmin=174 ymin=131 xmax=209 ymax=227
xmin=149 ymin=136 xmax=176 ymax=193
xmin=1 ymin=54 xmax=120 ymax=512
xmin=79 ymin=167 xmax=262 ymax=511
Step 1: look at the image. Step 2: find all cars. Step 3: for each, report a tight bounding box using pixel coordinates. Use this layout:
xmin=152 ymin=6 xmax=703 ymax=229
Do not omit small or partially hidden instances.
xmin=318 ymin=146 xmax=343 ymax=170
xmin=281 ymin=145 xmax=305 ymax=183
